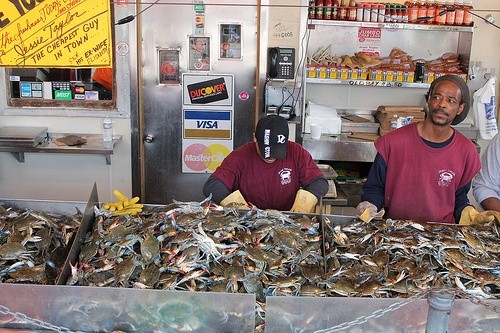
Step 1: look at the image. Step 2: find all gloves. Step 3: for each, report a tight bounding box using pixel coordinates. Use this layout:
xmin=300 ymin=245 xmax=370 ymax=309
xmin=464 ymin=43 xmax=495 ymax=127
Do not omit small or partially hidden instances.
xmin=220 ymin=189 xmax=249 ymax=206
xmin=458 ymin=206 xmax=500 ymax=226
xmin=290 ymin=190 xmax=318 ymax=213
xmin=103 ymin=189 xmax=144 ymax=216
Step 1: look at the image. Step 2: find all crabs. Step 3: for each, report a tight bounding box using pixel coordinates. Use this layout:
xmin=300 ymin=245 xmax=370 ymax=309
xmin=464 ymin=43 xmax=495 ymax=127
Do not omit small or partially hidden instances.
xmin=0 ymin=192 xmax=500 ymax=333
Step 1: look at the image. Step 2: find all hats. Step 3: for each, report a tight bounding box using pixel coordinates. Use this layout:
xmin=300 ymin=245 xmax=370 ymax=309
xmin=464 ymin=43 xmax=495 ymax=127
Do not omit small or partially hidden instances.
xmin=255 ymin=115 xmax=289 ymax=159
xmin=431 ymin=75 xmax=471 ymax=125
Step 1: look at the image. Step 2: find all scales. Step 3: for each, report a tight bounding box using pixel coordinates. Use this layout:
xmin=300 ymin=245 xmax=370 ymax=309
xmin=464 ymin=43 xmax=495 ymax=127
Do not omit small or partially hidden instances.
xmin=299 ymin=164 xmax=339 ymax=198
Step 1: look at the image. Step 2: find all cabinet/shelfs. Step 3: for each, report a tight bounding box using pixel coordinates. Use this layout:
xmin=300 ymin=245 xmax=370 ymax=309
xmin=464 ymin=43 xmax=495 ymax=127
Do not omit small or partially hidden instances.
xmin=302 ymin=0 xmax=473 ymax=162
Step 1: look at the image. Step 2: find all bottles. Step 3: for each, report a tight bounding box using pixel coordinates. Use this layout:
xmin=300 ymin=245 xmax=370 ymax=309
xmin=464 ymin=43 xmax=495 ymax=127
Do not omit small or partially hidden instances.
xmin=390 ymin=115 xmax=398 ymax=131
xmin=308 ymin=0 xmax=474 ymax=26
xmin=103 ymin=115 xmax=112 ymax=141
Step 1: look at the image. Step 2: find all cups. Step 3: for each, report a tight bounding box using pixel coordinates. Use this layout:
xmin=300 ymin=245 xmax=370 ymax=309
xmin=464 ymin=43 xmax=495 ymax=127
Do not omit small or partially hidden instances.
xmin=310 ymin=123 xmax=323 ymax=139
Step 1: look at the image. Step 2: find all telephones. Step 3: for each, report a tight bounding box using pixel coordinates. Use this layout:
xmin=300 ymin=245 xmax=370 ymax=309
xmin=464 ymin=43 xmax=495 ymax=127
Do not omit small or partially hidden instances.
xmin=267 ymin=47 xmax=295 ymax=79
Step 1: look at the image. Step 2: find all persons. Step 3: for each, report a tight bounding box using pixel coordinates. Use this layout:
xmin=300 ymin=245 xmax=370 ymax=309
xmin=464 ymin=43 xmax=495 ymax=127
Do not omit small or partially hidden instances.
xmin=472 ymin=131 xmax=500 ymax=212
xmin=356 ymin=74 xmax=494 ymax=226
xmin=202 ymin=115 xmax=329 ymax=213
